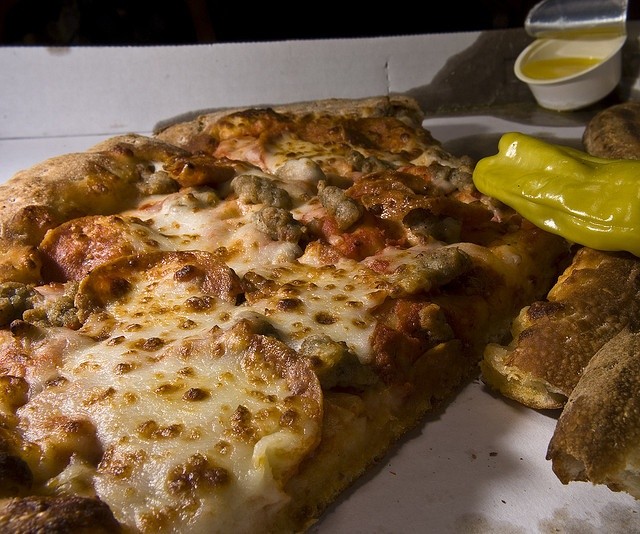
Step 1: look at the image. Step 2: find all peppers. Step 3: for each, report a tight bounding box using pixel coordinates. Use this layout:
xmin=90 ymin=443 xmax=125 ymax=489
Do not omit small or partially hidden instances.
xmin=474 ymin=135 xmax=639 ymax=261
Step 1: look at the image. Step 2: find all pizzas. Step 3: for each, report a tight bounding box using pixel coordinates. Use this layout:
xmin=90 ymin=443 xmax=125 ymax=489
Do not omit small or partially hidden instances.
xmin=0 ymin=92 xmax=639 ymax=534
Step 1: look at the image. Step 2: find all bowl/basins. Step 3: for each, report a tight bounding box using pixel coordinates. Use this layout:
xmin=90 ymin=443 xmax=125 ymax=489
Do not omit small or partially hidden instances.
xmin=513 ymin=35 xmax=628 ymax=113
